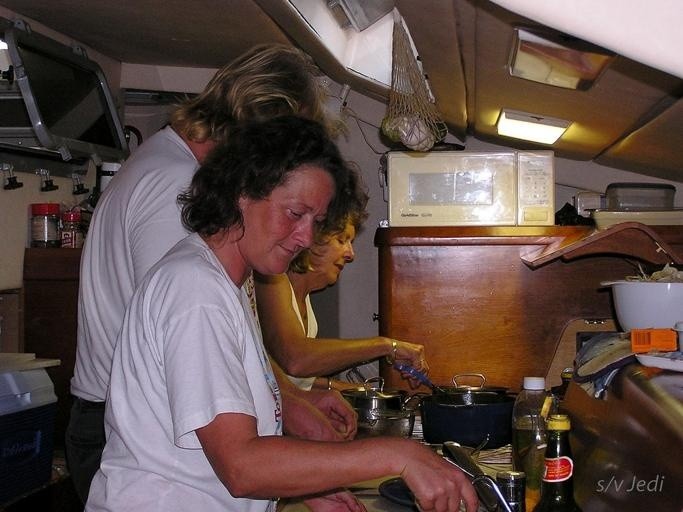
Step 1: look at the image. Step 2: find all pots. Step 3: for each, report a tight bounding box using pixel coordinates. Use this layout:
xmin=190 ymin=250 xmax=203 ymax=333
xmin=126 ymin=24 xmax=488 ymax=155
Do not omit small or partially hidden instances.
xmin=394 ymin=366 xmax=516 ymax=394
xmin=340 ymin=376 xmax=430 ymax=439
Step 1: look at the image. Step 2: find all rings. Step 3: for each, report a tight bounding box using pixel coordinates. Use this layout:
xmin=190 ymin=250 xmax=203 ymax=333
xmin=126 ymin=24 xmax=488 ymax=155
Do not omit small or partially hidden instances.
xmin=419 ymin=359 xmax=426 ymax=363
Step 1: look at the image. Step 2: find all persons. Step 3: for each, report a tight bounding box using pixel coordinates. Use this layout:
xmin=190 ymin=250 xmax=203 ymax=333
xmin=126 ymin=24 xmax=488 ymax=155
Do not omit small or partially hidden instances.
xmin=82 ymin=114 xmax=481 ymax=512
xmin=253 ymin=160 xmax=431 ymax=393
xmin=62 ymin=41 xmax=362 ymax=512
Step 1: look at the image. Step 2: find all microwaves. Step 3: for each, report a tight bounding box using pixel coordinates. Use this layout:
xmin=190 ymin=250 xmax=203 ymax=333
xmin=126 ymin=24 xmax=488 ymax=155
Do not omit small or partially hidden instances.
xmin=387 ymin=148 xmax=555 ymax=226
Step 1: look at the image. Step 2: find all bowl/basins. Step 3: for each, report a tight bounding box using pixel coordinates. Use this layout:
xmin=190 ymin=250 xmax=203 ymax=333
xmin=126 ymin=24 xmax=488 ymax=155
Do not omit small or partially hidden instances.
xmin=419 ymin=394 xmax=515 ymax=449
xmin=600 ymin=278 xmax=683 ymax=332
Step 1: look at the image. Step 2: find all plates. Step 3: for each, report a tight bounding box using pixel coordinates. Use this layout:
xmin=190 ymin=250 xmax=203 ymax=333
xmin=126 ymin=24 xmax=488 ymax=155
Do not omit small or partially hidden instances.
xmin=378 ymin=477 xmax=417 ymax=512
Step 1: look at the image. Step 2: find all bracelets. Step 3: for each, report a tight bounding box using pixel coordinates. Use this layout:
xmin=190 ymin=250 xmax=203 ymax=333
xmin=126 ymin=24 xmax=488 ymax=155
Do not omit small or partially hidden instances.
xmin=327 ymin=375 xmax=332 ymax=389
xmin=386 ymin=338 xmax=397 ymax=365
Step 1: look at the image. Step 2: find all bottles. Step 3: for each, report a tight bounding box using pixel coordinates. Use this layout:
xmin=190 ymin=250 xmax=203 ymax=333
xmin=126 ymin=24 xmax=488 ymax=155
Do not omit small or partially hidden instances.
xmin=29 ymin=203 xmax=83 ymax=249
xmin=99 ymin=160 xmax=121 ymax=193
xmin=496 ymin=377 xmax=582 ymax=512
